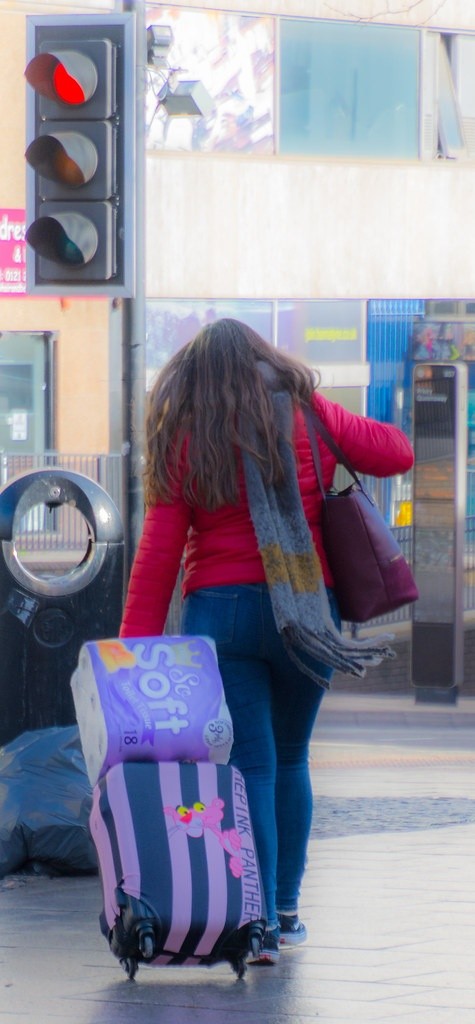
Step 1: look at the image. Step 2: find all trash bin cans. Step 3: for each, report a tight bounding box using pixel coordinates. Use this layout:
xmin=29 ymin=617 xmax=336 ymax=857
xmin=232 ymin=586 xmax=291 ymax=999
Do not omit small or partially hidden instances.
xmin=1 ymin=469 xmax=128 ymax=743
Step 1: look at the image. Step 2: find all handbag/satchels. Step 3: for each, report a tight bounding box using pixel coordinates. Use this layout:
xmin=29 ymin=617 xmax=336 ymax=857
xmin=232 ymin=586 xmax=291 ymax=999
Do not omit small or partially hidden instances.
xmin=300 ymin=398 xmax=419 ymax=622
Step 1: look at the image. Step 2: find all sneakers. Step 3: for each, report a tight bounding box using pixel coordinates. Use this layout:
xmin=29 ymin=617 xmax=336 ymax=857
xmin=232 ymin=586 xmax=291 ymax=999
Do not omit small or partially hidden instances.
xmin=277 ymin=913 xmax=307 ymax=946
xmin=246 ymin=925 xmax=280 ymax=965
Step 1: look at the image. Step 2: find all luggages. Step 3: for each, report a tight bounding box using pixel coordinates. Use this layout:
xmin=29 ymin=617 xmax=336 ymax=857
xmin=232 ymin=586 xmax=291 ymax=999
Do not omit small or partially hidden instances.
xmin=88 ymin=760 xmax=268 ymax=979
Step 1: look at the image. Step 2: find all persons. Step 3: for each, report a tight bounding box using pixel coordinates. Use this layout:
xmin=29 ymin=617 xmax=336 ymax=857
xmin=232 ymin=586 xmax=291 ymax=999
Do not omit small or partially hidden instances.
xmin=116 ymin=318 xmax=413 ymax=961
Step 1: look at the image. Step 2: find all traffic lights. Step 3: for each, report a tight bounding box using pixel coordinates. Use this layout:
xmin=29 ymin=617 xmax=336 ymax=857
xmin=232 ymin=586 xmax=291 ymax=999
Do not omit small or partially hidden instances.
xmin=24 ymin=9 xmax=137 ymax=298
